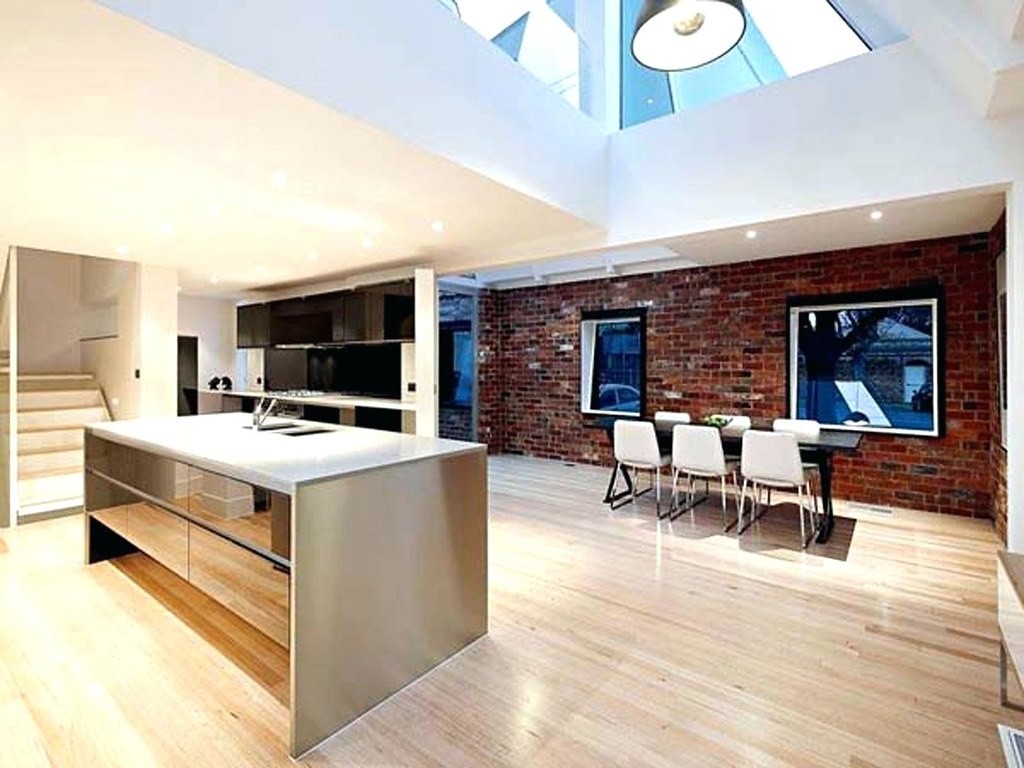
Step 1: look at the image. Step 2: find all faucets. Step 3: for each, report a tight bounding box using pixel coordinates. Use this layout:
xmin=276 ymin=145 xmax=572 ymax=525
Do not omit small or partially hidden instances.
xmin=251 ymin=394 xmax=280 ymax=433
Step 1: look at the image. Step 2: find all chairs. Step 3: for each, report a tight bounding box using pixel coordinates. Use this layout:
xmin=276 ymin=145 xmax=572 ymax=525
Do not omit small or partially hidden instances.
xmin=610 ymin=409 xmax=829 ymax=548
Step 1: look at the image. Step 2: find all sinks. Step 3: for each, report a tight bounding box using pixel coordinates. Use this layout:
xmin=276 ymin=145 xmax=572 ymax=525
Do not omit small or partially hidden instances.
xmin=278 ymin=427 xmax=339 ymax=439
xmin=242 ymin=422 xmax=302 ymax=434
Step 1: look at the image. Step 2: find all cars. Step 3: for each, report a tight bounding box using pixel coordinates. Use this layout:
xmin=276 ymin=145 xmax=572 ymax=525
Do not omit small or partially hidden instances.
xmin=911 ymin=382 xmax=933 ymax=412
xmin=598 ymin=383 xmax=639 ymax=414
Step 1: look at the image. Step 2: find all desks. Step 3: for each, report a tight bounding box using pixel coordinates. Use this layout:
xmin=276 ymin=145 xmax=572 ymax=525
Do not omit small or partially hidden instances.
xmin=583 ymin=418 xmax=863 ymax=545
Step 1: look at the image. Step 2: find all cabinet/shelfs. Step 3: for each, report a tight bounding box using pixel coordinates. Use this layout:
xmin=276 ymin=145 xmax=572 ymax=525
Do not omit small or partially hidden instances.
xmin=237 ymin=277 xmax=416 ymax=349
xmin=996 ymin=550 xmax=1024 ymax=705
xmin=78 ymin=413 xmax=489 ymax=759
